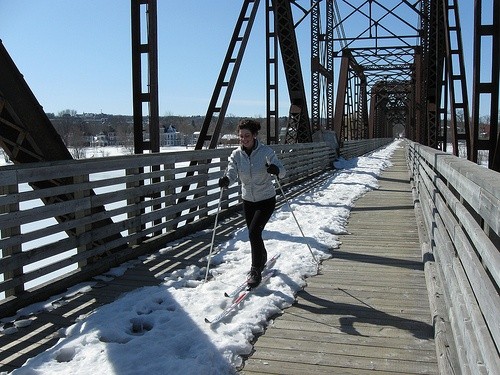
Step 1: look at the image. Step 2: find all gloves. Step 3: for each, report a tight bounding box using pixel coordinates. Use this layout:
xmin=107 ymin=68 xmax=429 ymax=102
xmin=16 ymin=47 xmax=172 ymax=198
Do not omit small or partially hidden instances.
xmin=265 ymin=163 xmax=279 ymax=175
xmin=219 ymin=176 xmax=229 ymax=186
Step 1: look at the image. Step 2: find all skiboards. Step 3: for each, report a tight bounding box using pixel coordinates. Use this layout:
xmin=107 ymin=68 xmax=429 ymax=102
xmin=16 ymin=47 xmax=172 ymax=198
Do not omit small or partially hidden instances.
xmin=205 ymin=251 xmax=281 ymax=324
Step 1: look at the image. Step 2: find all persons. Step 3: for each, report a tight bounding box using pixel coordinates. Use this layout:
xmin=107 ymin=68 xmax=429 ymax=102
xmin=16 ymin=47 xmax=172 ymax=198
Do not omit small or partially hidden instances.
xmin=218 ymin=120 xmax=287 ymax=287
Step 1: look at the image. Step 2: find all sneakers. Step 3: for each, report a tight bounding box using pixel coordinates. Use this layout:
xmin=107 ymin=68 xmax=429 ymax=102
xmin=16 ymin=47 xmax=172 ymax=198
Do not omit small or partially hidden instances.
xmin=262 ymin=252 xmax=267 ymax=265
xmin=247 ymin=266 xmax=262 ymax=287
